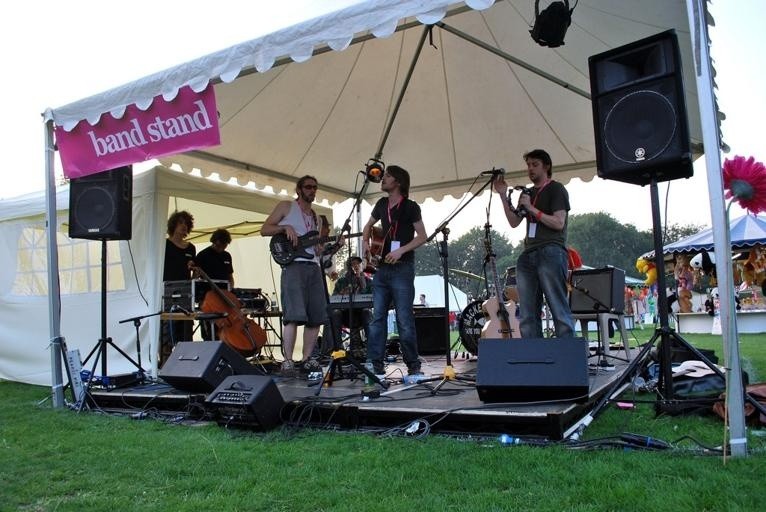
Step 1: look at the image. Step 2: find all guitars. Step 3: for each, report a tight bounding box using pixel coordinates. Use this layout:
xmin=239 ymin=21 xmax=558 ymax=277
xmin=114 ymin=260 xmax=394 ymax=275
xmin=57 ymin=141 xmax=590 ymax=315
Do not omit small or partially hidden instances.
xmin=270 ymin=231 xmax=364 ymax=265
xmin=481 ymin=239 xmax=523 ymax=338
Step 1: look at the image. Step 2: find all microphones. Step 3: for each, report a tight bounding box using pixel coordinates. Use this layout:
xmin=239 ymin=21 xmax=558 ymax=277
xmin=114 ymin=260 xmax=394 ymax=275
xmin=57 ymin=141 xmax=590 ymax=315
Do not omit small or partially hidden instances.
xmin=362 ymin=171 xmax=380 ymax=183
xmin=324 ymin=244 xmax=335 ymax=249
xmin=481 ymin=168 xmax=505 ymax=175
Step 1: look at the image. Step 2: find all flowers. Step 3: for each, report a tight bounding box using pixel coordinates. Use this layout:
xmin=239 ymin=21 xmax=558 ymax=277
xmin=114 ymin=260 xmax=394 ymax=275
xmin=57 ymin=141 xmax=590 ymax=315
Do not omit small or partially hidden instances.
xmin=719 ymin=151 xmax=766 ymax=231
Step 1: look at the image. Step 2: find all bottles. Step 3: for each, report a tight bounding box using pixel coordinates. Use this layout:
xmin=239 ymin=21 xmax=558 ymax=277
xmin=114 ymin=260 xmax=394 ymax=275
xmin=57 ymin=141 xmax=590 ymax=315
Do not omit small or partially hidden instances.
xmin=363 ymin=358 xmax=376 ymax=387
xmin=271 ymin=292 xmax=277 ymax=311
xmin=402 ymin=375 xmax=432 ymax=385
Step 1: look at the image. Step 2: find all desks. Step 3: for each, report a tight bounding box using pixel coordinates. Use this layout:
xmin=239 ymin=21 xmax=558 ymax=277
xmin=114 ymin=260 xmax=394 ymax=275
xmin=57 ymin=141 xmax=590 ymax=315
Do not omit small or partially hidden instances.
xmin=159 ymin=308 xmax=284 ymax=369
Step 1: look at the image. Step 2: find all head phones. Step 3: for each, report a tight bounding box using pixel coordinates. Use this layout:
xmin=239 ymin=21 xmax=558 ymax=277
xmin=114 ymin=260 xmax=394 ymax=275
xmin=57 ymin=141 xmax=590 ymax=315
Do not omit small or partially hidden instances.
xmin=508 ymin=185 xmax=531 ymax=217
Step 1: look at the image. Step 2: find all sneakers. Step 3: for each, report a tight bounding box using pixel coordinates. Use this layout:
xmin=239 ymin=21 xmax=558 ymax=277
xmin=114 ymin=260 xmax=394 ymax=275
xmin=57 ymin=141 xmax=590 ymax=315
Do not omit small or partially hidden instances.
xmin=281 ymin=359 xmax=296 ymax=378
xmin=300 ymin=356 xmax=323 ymax=380
xmin=362 ymin=361 xmax=386 ymax=377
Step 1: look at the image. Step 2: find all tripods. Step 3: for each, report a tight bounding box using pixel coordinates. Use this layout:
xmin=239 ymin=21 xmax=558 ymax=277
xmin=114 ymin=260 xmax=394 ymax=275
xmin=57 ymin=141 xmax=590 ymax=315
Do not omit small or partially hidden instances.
xmin=315 ymin=252 xmax=388 ymax=395
xmin=308 ymin=180 xmax=365 ymax=387
xmin=570 ymin=175 xmax=766 ymax=444
xmin=36 ymin=241 xmax=146 ymax=414
xmin=570 ymin=284 xmax=630 ymax=377
xmin=416 ymin=176 xmax=495 ymax=394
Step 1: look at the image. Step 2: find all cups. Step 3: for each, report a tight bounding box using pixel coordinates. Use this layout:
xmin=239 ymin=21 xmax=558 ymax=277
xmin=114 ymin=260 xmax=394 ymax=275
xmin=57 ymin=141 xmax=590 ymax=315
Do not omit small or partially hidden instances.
xmin=271 ymin=306 xmax=278 ymax=312
xmin=322 ymin=366 xmax=329 ymax=381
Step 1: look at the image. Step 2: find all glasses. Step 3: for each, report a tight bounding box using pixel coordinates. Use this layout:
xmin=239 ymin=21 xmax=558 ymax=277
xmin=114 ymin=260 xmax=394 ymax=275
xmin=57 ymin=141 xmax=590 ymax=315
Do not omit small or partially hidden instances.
xmin=303 ymin=185 xmax=318 ymax=190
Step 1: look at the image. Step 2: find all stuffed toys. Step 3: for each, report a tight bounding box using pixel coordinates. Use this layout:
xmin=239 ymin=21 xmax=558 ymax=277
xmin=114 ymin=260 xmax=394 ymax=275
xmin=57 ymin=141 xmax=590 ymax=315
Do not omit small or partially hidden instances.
xmin=624 ymin=250 xmax=766 ymax=313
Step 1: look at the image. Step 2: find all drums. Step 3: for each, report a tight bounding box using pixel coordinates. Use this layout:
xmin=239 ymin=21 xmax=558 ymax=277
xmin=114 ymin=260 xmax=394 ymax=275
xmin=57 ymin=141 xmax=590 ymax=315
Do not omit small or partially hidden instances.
xmin=503 ymin=265 xmax=519 ymax=305
xmin=459 ymin=301 xmax=519 ymax=355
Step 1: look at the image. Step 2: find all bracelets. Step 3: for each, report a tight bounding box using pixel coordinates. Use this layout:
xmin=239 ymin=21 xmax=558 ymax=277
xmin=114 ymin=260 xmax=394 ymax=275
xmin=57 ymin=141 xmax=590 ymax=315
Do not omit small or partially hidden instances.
xmin=536 ymin=210 xmax=542 ymax=221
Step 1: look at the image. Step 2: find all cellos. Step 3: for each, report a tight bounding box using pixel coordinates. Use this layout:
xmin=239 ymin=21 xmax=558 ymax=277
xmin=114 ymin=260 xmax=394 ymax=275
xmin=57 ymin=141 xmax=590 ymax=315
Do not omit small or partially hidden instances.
xmin=190 ymin=264 xmax=267 ymax=357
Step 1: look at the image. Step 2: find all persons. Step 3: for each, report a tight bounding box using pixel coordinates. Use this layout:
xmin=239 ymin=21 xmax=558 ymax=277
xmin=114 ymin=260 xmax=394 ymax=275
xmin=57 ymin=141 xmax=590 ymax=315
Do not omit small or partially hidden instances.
xmin=317 ymin=214 xmax=344 ymax=351
xmin=191 ymin=228 xmax=234 ymax=341
xmin=159 ymin=210 xmax=195 ymax=366
xmin=330 ymin=257 xmax=372 ymax=353
xmin=493 ymin=149 xmax=576 ymax=339
xmin=363 ymin=165 xmax=427 ymax=377
xmin=417 ymin=294 xmax=427 ymax=307
xmin=259 ymin=173 xmax=323 ymax=371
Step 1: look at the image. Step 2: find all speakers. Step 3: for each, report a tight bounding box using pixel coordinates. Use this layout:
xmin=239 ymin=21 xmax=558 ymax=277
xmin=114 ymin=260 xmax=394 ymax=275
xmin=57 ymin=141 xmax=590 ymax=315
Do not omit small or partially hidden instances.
xmin=476 ymin=337 xmax=589 ymax=403
xmin=412 ymin=307 xmax=446 ymax=355
xmin=588 ymin=28 xmax=693 ymax=187
xmin=569 ymin=266 xmax=625 ymax=314
xmin=68 ymin=165 xmax=132 ymax=240
xmin=157 ymin=340 xmax=267 ymax=394
xmin=205 ymin=374 xmax=286 ymax=434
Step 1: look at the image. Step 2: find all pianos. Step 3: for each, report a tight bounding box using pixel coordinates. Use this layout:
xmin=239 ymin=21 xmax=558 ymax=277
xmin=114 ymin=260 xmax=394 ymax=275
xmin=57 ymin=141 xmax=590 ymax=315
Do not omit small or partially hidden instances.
xmin=329 ymin=292 xmax=374 ymax=309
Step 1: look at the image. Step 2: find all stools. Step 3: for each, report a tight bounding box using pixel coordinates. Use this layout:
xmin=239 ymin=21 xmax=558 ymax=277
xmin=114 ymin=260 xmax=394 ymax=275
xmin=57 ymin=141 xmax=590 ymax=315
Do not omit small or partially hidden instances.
xmin=567 ymin=311 xmax=631 ymax=363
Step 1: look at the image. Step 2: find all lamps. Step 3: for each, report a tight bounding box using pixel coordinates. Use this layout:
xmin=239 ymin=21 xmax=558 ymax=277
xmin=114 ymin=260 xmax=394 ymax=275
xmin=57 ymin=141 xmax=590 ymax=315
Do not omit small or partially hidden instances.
xmin=526 ymin=1 xmax=581 ymax=51
